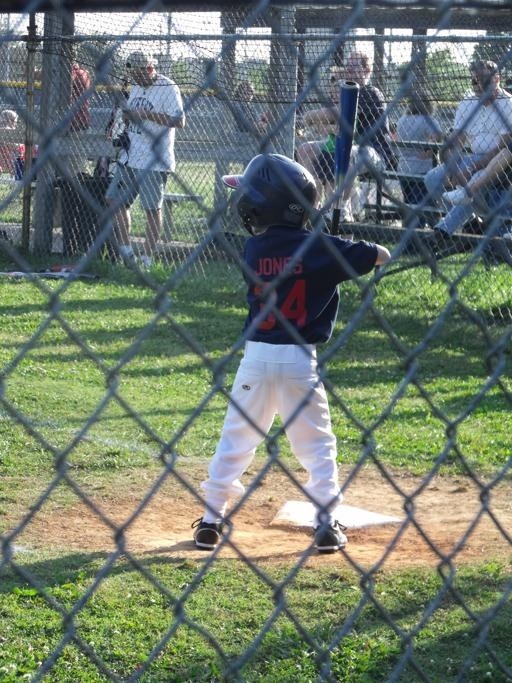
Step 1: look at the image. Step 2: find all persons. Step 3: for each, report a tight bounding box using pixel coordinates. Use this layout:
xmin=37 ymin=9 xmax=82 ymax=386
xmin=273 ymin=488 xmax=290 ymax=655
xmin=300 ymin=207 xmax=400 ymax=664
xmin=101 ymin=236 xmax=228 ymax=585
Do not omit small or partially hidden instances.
xmin=234 ymin=80 xmax=269 ymax=136
xmin=335 ymin=52 xmax=512 ymax=236
xmin=191 ymin=151 xmax=392 ymax=555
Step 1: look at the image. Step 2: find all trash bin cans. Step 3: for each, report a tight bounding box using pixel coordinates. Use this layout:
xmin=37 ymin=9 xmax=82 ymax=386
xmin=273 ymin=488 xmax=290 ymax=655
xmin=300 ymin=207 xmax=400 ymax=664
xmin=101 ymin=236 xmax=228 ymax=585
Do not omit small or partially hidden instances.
xmin=59 ymin=176 xmax=116 ymax=259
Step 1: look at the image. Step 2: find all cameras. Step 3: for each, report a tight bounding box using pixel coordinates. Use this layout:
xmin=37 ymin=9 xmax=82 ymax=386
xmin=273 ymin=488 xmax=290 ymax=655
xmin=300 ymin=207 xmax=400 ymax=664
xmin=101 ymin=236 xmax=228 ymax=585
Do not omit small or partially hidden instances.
xmin=112 ymin=134 xmax=131 ymax=151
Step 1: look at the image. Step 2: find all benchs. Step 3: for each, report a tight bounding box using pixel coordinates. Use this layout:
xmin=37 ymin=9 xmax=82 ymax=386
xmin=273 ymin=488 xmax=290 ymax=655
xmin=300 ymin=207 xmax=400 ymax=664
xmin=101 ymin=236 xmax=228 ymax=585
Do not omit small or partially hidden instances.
xmin=152 ymin=187 xmax=204 ymax=241
xmin=317 ymin=134 xmax=511 ymax=255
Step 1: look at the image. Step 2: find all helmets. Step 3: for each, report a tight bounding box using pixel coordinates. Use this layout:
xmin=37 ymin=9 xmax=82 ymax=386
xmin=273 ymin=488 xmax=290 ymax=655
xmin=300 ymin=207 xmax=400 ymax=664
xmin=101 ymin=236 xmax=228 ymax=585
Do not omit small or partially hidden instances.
xmin=222 ymin=153 xmax=317 ymax=229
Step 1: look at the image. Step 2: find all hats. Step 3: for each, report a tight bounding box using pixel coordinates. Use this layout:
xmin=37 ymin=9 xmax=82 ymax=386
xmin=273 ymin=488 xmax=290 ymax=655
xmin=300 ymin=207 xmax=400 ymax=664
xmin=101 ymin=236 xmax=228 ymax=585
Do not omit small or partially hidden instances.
xmin=470 ymin=58 xmax=499 ymax=73
xmin=124 ymin=50 xmax=159 ymax=71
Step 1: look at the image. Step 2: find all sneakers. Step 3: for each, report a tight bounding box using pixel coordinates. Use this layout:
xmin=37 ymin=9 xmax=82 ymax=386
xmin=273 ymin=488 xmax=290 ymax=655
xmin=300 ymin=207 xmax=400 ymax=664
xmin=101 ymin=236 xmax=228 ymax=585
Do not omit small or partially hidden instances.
xmin=323 ymin=194 xmax=355 ymax=225
xmin=431 ymin=218 xmax=447 ymax=240
xmin=189 ymin=517 xmax=225 ymax=549
xmin=313 ymin=519 xmax=351 ymax=555
xmin=443 ymin=186 xmax=476 ymax=206
xmin=115 ymin=244 xmax=155 ymax=270
xmin=500 ymin=223 xmax=511 ymax=240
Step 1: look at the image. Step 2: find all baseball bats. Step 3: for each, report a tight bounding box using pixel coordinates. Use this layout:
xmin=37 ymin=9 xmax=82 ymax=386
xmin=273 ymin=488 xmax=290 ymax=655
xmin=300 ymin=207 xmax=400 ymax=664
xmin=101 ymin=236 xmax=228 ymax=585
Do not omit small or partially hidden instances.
xmin=330 ymin=79 xmax=360 ymax=238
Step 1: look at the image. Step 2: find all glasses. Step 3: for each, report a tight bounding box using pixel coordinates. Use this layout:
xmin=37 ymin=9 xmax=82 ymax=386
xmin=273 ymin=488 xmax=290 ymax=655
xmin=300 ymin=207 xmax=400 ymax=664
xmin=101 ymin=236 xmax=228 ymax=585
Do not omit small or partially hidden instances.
xmin=470 ymin=77 xmax=498 ymax=84
